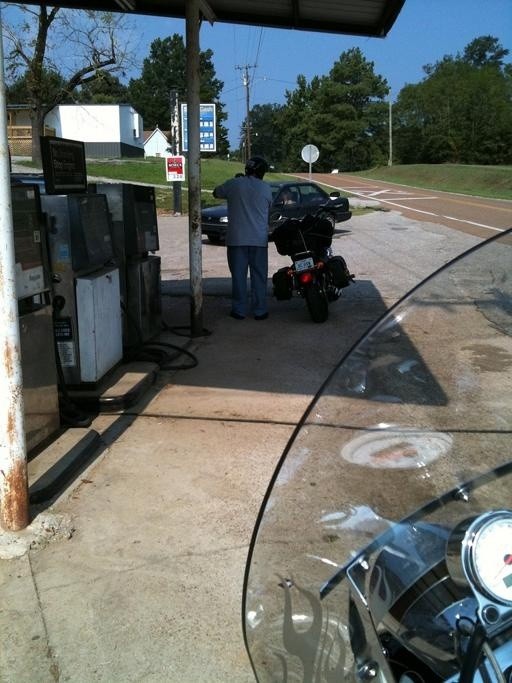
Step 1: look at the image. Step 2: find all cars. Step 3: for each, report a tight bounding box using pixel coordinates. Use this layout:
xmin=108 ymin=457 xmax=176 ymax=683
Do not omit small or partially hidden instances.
xmin=201 ymin=181 xmax=353 ymax=246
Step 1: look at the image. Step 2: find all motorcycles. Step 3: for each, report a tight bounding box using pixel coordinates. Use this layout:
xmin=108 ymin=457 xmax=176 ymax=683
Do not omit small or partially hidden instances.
xmin=239 ymin=225 xmax=511 ymax=683
xmin=263 ymin=180 xmax=356 ymax=322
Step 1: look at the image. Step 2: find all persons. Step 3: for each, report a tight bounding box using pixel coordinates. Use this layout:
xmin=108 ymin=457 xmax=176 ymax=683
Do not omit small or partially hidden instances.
xmin=213 ymin=156 xmax=274 ymax=320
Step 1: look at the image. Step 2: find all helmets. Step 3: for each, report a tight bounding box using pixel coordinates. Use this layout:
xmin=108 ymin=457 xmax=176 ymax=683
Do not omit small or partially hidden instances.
xmin=245 ymin=157 xmax=268 ymax=178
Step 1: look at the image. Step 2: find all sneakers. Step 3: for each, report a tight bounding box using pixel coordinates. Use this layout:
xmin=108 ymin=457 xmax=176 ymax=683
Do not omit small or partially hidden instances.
xmin=230 ymin=310 xmax=245 ymax=320
xmin=255 ymin=310 xmax=270 ymax=320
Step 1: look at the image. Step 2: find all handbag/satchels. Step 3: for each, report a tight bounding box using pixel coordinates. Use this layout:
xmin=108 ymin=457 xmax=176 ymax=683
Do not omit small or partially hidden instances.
xmin=328 ymin=256 xmax=349 ymax=288
xmin=272 ymin=267 xmax=293 ymax=301
xmin=273 ymin=216 xmax=334 ymax=255
